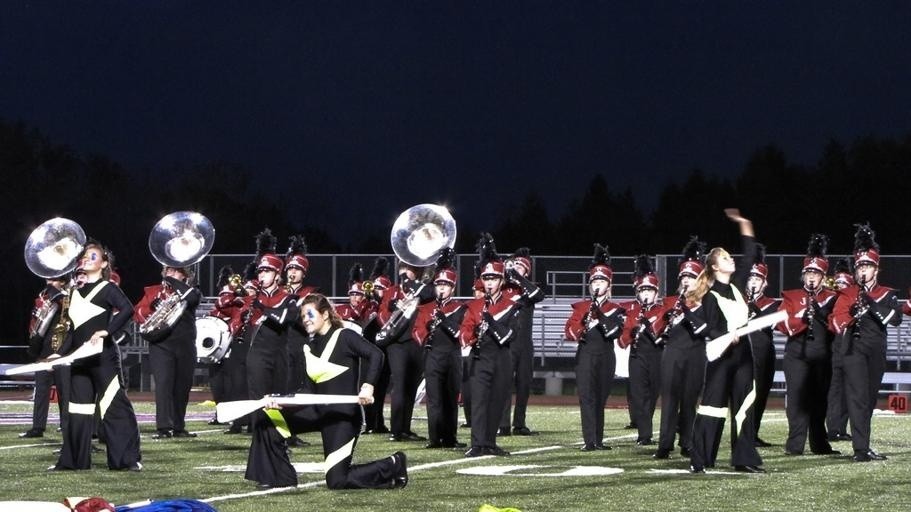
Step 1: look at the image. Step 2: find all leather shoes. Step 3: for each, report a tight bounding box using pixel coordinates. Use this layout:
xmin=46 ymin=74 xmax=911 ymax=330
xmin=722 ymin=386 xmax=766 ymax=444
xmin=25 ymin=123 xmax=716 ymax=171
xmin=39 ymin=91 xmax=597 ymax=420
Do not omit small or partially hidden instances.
xmin=829 ymin=432 xmax=886 ymax=462
xmin=361 ymin=427 xmax=426 ymax=442
xmin=391 ymin=449 xmax=408 ymax=490
xmin=174 ymin=430 xmax=196 ymax=438
xmin=425 ymin=436 xmax=511 ymax=458
xmin=580 ymin=444 xmax=595 ymax=451
xmin=622 ymin=422 xmax=705 ymax=473
xmin=496 ymin=426 xmax=511 ymax=437
xmin=512 ymin=426 xmax=530 ymax=436
xmin=207 ymin=417 xmax=229 ymax=425
xmin=151 ymin=431 xmax=172 ymax=439
xmin=289 ymin=438 xmax=311 ymax=446
xmin=595 ymin=443 xmax=611 ymax=450
xmin=223 ymin=427 xmax=242 ymax=434
xmin=53 ymin=425 xmax=104 ymax=456
xmin=460 ymin=423 xmax=470 ymax=427
xmin=735 ymin=464 xmax=766 ymax=474
xmin=754 ymin=437 xmax=771 ymax=447
xmin=18 ymin=430 xmax=43 ymax=438
xmin=256 ymin=481 xmax=270 ymax=489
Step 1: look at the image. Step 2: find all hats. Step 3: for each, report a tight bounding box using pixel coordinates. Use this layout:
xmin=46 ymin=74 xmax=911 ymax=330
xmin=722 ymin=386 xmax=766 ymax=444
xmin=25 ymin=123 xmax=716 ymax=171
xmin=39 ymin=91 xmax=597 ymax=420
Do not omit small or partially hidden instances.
xmin=801 ymin=233 xmax=829 ymax=275
xmin=368 ymin=258 xmax=391 ymax=289
xmin=432 ymin=247 xmax=457 ymax=285
xmin=677 ymin=236 xmax=705 ymax=278
xmin=832 ymin=259 xmax=854 ymax=286
xmin=218 ymin=230 xmax=309 ymax=296
xmin=587 ymin=242 xmax=613 ymax=284
xmin=747 ymin=245 xmax=769 ymax=281
xmin=852 ymin=221 xmax=881 ymax=266
xmin=470 ymin=231 xmax=532 ymax=289
xmin=631 ymin=254 xmax=659 ymax=291
xmin=347 ymin=262 xmax=365 ymax=294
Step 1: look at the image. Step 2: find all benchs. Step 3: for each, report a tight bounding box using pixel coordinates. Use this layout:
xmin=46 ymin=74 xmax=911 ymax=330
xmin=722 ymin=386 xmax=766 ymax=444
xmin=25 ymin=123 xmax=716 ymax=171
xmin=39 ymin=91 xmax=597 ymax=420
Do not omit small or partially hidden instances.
xmin=187 ymin=296 xmax=911 ymax=366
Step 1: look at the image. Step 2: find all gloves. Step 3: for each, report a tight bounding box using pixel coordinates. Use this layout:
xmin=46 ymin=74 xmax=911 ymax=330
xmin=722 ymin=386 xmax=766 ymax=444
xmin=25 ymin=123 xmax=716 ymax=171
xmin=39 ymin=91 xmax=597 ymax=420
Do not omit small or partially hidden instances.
xmin=849 ymin=304 xmax=858 ymax=316
xmin=435 ymin=311 xmax=446 ymax=320
xmin=249 ymin=299 xmax=264 ymax=311
xmin=239 ymin=310 xmax=248 ymax=323
xmin=589 ymin=303 xmax=602 ymax=317
xmin=425 ymin=320 xmax=434 ymax=331
xmin=630 ymin=326 xmax=640 ymax=338
xmin=810 ymin=298 xmax=820 ymax=312
xmin=639 ymin=316 xmax=650 ymax=327
xmin=162 ymin=276 xmax=174 ymax=286
xmin=861 ymin=292 xmax=873 ymax=305
xmin=802 ymin=308 xmax=810 ymax=326
xmin=480 ymin=312 xmax=494 ymax=325
xmin=748 ymin=303 xmax=762 ymax=316
xmin=679 ymin=300 xmax=690 ymax=313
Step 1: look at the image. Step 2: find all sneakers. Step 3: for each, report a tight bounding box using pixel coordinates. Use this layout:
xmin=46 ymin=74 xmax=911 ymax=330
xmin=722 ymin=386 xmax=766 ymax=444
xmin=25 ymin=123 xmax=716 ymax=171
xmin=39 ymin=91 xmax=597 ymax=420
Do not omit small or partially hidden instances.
xmin=46 ymin=464 xmax=56 ymax=471
xmin=128 ymin=461 xmax=142 ymax=471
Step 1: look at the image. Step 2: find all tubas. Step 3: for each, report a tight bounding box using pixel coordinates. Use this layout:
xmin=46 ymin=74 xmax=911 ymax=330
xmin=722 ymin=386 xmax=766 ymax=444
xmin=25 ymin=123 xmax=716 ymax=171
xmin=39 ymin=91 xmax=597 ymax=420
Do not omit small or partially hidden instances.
xmin=24 ymin=217 xmax=86 ymax=352
xmin=374 ymin=203 xmax=458 ymax=342
xmin=138 ymin=210 xmax=216 ymax=334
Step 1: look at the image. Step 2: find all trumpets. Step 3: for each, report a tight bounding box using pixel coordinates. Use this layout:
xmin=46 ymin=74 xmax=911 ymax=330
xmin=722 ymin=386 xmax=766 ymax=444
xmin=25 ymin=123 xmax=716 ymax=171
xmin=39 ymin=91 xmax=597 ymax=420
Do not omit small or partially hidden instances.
xmin=227 ymin=274 xmax=249 ymax=300
xmin=361 ymin=281 xmax=374 ymax=299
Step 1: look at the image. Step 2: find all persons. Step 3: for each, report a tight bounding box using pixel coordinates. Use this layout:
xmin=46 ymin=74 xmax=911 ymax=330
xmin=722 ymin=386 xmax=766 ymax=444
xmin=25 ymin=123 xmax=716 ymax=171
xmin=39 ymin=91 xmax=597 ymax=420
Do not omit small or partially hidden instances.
xmin=568 ymin=211 xmax=903 ymax=473
xmin=17 ymin=237 xmax=541 ymax=488
